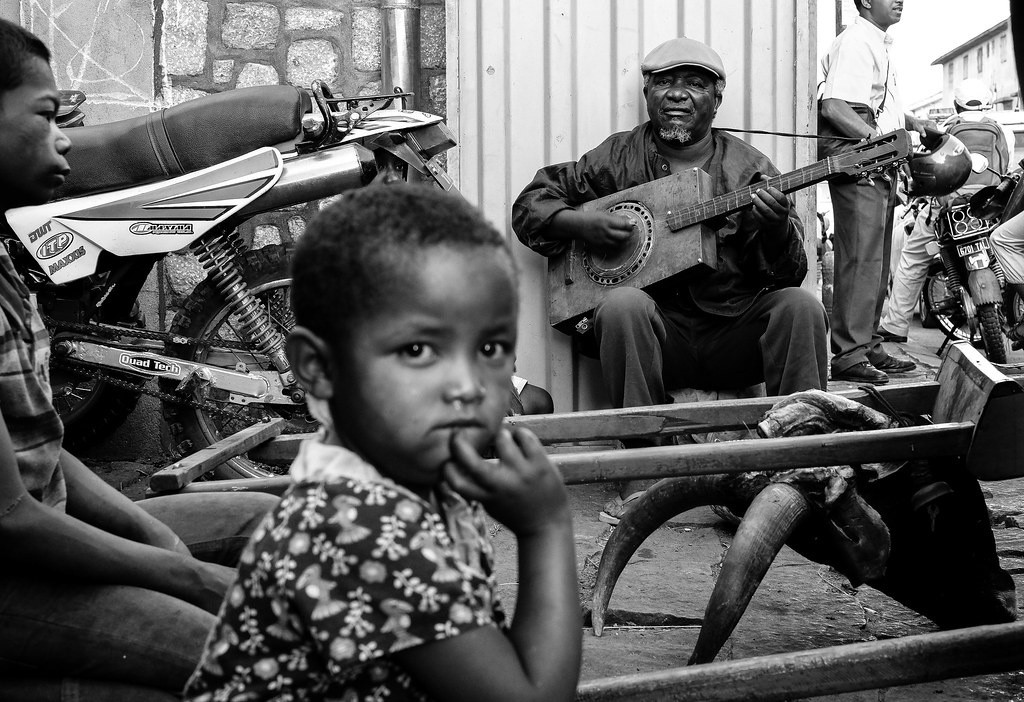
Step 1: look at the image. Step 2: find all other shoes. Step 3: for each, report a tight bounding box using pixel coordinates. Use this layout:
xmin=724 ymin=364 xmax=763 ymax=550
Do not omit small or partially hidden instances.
xmin=1006 ymin=320 xmax=1024 ymax=341
xmin=875 ymin=325 xmax=909 ymax=342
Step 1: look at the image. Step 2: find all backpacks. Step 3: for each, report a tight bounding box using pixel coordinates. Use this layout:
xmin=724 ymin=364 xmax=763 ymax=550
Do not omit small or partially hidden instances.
xmin=944 ymin=118 xmax=1009 ymax=199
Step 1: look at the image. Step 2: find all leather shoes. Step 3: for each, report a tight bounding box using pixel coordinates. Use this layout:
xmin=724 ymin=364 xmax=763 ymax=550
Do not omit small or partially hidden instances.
xmin=832 ymin=362 xmax=891 ymax=384
xmin=875 ymin=354 xmax=917 ymax=373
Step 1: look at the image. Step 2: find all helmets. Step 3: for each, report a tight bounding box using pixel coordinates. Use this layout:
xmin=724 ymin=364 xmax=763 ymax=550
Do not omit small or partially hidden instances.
xmin=907 ymin=129 xmax=973 ymax=198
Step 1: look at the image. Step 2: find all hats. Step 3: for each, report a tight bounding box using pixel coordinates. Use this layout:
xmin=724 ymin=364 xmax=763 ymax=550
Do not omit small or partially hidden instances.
xmin=641 ymin=36 xmax=728 ymax=81
xmin=954 ymin=82 xmax=992 ymax=111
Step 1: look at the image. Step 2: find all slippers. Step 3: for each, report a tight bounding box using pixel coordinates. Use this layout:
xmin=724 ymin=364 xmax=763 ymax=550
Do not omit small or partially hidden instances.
xmin=598 ymin=487 xmax=649 ymax=526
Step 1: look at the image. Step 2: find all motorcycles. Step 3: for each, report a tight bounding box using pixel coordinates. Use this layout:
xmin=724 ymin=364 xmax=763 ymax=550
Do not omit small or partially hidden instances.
xmin=894 ymin=158 xmax=1006 ymax=363
xmin=0 ymin=79 xmax=462 ymax=484
xmin=965 ymin=151 xmax=1024 ymax=352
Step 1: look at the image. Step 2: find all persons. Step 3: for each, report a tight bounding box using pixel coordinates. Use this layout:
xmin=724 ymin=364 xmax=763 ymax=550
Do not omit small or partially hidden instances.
xmin=178 ymin=182 xmax=583 ymax=701
xmin=510 ymin=36 xmax=831 ymax=525
xmin=871 ymin=74 xmax=1015 ymax=343
xmin=0 ymin=11 xmax=286 ymax=691
xmin=988 ymin=206 xmax=1024 ymax=346
xmin=815 ymin=1 xmax=942 ymax=386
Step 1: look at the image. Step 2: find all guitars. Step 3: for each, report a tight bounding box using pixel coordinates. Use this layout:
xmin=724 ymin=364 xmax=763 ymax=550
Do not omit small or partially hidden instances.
xmin=546 ymin=129 xmax=911 ymax=338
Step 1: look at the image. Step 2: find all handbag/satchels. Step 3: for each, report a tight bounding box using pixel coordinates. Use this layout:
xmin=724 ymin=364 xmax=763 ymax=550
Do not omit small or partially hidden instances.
xmin=816 ymin=102 xmax=877 ymax=182
